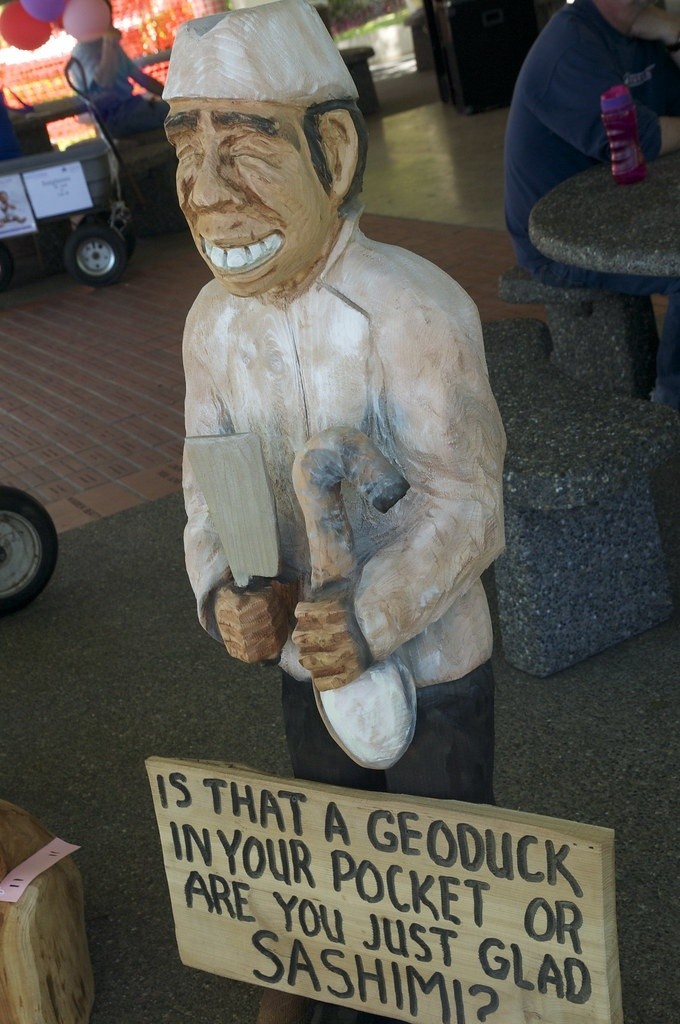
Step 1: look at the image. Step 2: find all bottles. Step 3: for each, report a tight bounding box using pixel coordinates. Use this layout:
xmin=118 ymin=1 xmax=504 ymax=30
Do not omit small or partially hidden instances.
xmin=601 ymin=82 xmax=647 ymax=185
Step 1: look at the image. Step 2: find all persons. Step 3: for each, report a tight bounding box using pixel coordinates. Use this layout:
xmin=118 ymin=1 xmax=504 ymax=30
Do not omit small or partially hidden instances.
xmin=505 ymin=0 xmax=680 ymax=401
xmin=164 ymin=1 xmax=506 ymax=1020
xmin=67 ymin=0 xmax=168 ymax=136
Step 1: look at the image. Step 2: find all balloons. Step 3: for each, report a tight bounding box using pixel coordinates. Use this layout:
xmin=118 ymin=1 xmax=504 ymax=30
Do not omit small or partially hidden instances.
xmin=0 ymin=0 xmax=111 ymax=49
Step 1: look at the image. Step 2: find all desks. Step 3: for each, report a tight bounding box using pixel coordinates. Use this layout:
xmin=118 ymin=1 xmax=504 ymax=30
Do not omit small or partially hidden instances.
xmin=528 ymin=151 xmax=680 ymax=399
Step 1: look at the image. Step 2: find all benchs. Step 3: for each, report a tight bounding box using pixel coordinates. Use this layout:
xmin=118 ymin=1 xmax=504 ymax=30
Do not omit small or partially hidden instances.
xmin=497 ymin=265 xmax=659 ymax=396
xmin=483 ymin=317 xmax=680 ymax=678
